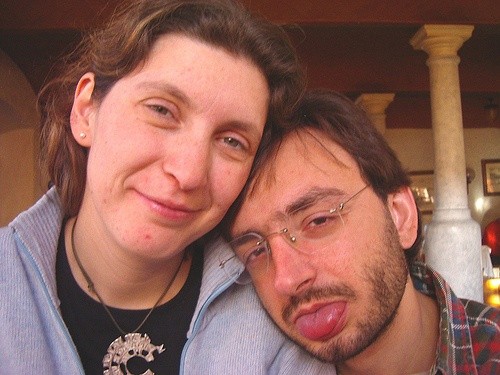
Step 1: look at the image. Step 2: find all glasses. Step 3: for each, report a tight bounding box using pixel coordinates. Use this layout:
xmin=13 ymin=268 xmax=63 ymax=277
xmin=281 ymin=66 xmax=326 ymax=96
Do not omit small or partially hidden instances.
xmin=217 ymin=184 xmax=372 ymax=285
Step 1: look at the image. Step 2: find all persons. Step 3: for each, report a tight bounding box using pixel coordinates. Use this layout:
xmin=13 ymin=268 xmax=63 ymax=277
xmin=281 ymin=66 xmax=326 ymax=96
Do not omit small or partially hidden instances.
xmin=215 ymin=81 xmax=500 ymax=373
xmin=0 ymin=1 xmax=340 ymax=373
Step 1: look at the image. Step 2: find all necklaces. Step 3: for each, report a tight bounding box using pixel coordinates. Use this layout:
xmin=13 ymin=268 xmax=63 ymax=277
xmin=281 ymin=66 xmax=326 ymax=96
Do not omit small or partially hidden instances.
xmin=67 ymin=210 xmax=205 ymax=375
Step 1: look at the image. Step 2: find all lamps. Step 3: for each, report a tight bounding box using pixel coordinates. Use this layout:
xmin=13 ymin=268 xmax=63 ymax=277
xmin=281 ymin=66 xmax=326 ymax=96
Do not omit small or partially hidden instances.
xmin=466 ymin=167 xmax=475 ymax=185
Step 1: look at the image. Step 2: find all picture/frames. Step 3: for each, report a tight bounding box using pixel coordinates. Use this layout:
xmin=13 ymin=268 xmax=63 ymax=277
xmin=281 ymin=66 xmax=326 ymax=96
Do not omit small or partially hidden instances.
xmin=481 ymin=158 xmax=500 ymax=197
xmin=407 ymin=170 xmax=436 ymax=216
